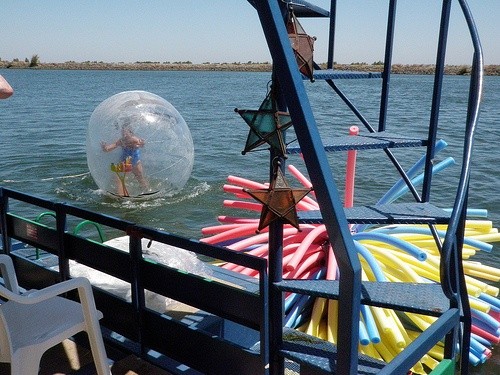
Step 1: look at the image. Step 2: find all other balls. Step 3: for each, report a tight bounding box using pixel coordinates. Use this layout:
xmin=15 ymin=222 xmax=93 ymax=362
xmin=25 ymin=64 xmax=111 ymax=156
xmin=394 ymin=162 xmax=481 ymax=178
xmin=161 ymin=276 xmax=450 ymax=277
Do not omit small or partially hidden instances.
xmin=85 ymin=89 xmax=195 ymax=200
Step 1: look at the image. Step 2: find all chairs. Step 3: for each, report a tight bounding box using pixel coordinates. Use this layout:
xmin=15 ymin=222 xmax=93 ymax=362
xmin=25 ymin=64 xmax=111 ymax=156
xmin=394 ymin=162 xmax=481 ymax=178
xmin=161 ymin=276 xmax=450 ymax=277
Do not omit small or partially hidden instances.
xmin=0 ymin=253 xmax=113 ymax=375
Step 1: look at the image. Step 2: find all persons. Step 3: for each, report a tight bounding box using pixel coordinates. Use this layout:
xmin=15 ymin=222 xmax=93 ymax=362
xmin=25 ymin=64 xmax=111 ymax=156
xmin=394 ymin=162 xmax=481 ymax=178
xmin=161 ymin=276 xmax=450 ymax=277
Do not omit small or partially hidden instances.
xmin=100 ymin=123 xmax=150 ymax=197
